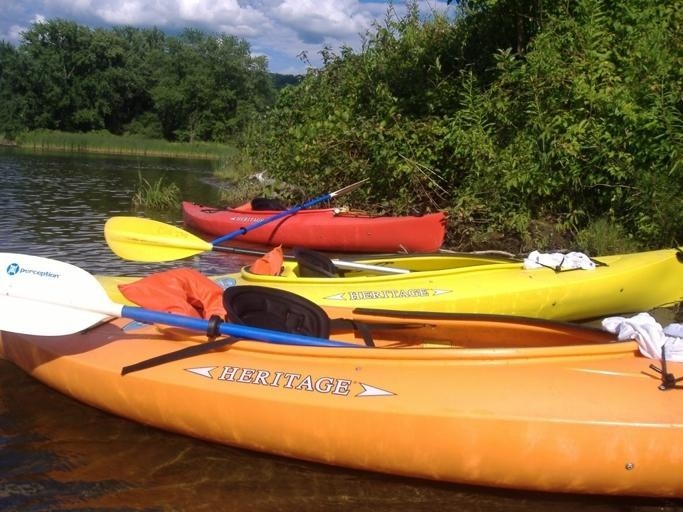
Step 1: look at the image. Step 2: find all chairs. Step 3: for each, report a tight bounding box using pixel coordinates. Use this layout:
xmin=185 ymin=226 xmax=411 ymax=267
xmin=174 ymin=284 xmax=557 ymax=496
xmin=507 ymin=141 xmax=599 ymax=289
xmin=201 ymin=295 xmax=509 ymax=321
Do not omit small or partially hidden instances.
xmin=294 ymin=247 xmax=351 ymax=277
xmin=250 ymin=198 xmax=286 ymax=211
xmin=219 ymin=285 xmax=376 ymax=348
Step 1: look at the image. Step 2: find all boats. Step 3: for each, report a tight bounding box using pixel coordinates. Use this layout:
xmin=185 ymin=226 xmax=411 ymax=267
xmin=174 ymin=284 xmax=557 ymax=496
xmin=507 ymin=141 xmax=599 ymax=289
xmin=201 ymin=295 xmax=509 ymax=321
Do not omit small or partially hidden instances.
xmin=179 ymin=196 xmax=448 ymax=255
xmin=0 ymin=296 xmax=682 ymax=503
xmin=89 ymin=242 xmax=682 ymax=335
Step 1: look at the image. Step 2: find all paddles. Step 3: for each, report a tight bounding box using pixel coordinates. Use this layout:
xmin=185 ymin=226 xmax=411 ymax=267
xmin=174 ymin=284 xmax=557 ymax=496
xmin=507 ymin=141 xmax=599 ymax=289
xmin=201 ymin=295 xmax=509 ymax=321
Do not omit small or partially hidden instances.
xmin=212 ymin=177 xmax=370 ymax=246
xmin=104 ymin=216 xmax=411 ymax=274
xmin=0 ymin=253 xmax=368 ymax=347
